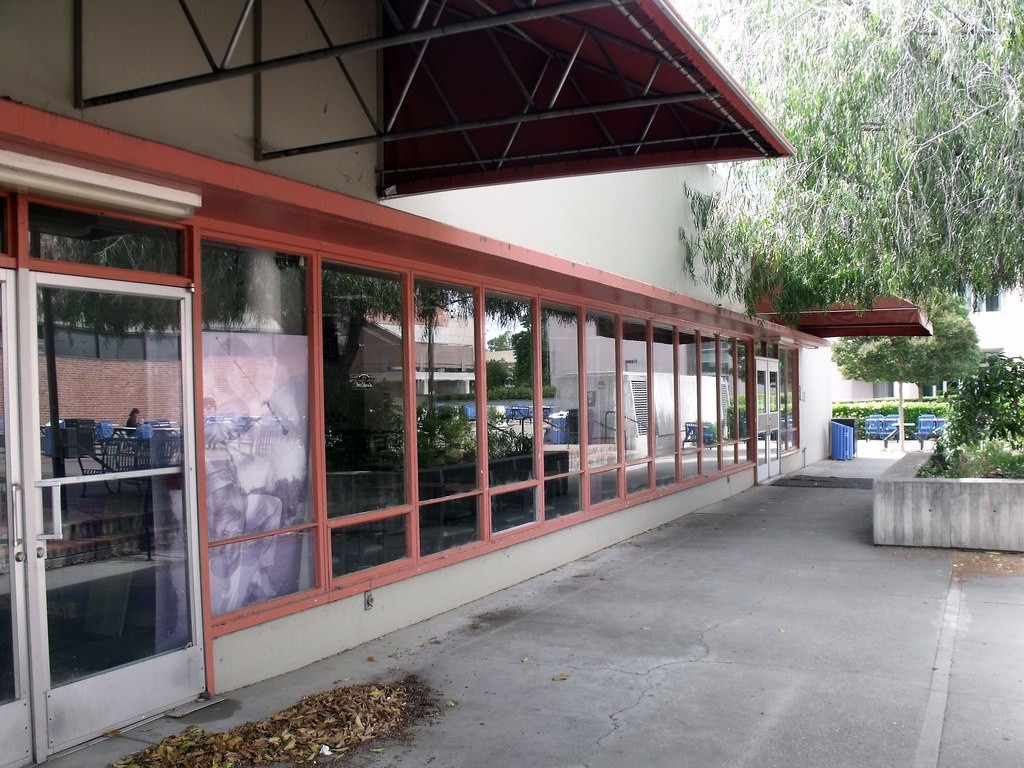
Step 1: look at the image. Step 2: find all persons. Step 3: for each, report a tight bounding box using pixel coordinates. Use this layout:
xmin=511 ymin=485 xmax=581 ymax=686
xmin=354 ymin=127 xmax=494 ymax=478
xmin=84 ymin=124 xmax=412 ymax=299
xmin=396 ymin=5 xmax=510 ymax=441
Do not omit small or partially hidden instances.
xmin=203 ymin=388 xmax=282 ymax=618
xmin=126 ymin=408 xmax=140 ymax=437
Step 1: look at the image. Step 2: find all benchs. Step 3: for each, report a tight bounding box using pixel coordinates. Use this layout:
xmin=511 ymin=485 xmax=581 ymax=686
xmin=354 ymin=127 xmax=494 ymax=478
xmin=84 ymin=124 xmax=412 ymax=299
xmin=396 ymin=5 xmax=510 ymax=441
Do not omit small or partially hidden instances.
xmin=682 ymin=421 xmax=713 ymax=449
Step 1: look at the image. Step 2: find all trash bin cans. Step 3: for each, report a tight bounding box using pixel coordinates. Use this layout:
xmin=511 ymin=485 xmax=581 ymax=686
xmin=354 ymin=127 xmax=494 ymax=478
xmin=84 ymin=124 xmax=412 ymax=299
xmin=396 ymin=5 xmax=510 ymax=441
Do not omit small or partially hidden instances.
xmin=830 ymin=420 xmax=854 ymax=460
xmin=61 ymin=416 xmax=95 ymax=458
xmin=550 ymin=414 xmax=567 ymax=444
xmin=831 ymin=419 xmax=856 ymax=458
xmin=568 ymin=409 xmax=579 ymax=444
xmin=45 ymin=420 xmax=64 ymax=457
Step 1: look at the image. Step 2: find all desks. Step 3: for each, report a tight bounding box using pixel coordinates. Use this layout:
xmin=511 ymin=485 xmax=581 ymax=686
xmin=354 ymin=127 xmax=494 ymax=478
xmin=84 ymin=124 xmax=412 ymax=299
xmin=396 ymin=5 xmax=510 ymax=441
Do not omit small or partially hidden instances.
xmin=157 ymin=421 xmax=176 ymax=428
xmin=152 ymin=428 xmax=181 ymax=435
xmin=153 ymin=424 xmax=169 ymax=427
xmin=92 ymin=424 xmax=119 ymax=437
xmin=112 ymin=426 xmax=137 ymax=438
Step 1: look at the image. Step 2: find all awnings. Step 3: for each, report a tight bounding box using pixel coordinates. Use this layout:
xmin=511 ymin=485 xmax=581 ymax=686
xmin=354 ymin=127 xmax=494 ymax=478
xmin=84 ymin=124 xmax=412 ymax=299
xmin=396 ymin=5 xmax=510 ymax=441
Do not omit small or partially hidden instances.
xmin=0 ymin=0 xmax=798 ymax=205
xmin=750 ymin=247 xmax=934 ymax=336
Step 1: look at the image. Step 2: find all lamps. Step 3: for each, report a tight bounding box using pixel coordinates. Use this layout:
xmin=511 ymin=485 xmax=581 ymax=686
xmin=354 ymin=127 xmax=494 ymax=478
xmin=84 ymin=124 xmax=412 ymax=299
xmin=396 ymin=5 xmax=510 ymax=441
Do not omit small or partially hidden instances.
xmin=1 ymin=149 xmax=204 ymax=222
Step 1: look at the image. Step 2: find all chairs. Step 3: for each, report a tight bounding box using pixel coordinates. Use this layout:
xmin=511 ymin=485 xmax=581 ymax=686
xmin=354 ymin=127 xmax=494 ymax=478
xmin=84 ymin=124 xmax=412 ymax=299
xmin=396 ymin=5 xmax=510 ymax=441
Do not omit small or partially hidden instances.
xmin=39 ymin=418 xmax=182 ymax=499
xmin=865 ymin=414 xmax=949 ymax=440
